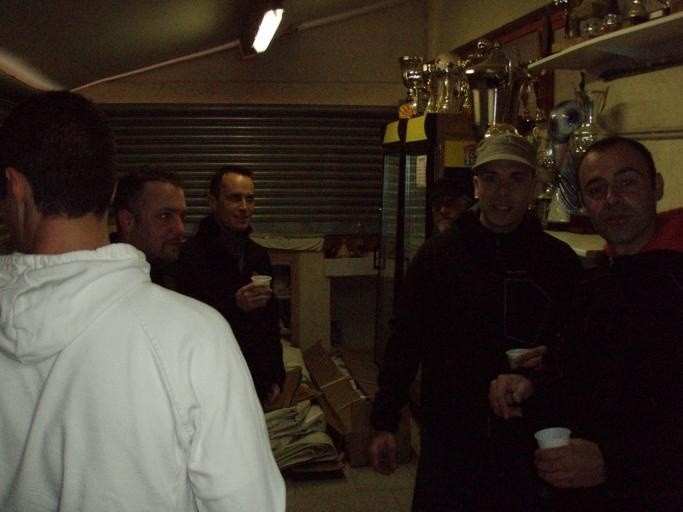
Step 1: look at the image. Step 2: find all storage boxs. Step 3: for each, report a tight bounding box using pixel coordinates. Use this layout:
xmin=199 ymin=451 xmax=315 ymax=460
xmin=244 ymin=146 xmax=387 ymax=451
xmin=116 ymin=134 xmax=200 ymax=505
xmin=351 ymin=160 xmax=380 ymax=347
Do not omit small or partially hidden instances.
xmin=304 ymin=341 xmax=411 ymax=470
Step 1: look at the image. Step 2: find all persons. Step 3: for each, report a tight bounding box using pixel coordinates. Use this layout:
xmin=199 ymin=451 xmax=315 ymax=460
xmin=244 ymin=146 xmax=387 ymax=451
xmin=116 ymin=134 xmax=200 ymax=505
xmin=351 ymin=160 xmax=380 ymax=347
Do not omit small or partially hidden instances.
xmin=0 ymin=89 xmax=288 ymax=512
xmin=111 ymin=161 xmax=200 ymax=300
xmin=485 ymin=133 xmax=683 ymax=510
xmin=424 ymin=169 xmax=475 ymax=236
xmin=364 ymin=131 xmax=584 ymax=511
xmin=174 ymin=163 xmax=287 ymax=414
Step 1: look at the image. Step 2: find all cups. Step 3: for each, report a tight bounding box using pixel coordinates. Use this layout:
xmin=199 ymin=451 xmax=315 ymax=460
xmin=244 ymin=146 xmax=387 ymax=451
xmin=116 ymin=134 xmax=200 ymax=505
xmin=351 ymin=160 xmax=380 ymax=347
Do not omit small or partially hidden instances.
xmin=535 ymin=427 xmax=571 ymax=451
xmin=251 ymin=275 xmax=272 ymax=289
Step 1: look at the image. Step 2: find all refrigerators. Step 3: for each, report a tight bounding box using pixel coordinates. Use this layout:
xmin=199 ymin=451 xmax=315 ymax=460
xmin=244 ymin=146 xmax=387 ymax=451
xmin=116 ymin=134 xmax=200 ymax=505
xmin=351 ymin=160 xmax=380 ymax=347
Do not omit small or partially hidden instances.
xmin=373 ymin=112 xmax=475 ymax=364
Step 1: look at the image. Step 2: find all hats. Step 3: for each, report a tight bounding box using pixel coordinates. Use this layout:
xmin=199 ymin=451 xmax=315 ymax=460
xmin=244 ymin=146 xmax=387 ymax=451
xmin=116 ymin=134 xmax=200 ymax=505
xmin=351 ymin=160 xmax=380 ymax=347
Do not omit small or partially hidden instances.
xmin=471 ymin=133 xmax=538 ymax=172
xmin=428 ymin=173 xmax=474 ymax=201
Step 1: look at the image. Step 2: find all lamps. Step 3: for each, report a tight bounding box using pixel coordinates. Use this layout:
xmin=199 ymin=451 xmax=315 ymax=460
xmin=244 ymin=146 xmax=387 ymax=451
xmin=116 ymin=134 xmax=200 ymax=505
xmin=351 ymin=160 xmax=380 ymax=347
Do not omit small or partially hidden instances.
xmin=239 ymin=0 xmax=286 ymax=61
